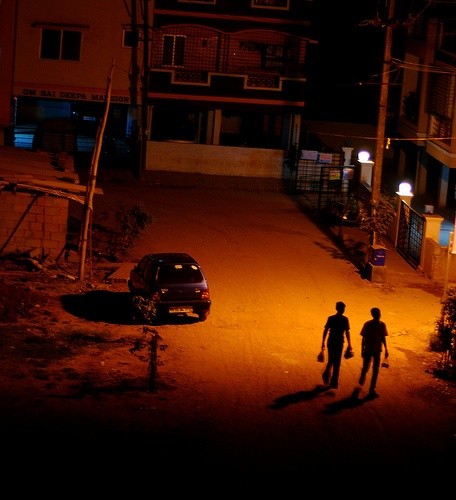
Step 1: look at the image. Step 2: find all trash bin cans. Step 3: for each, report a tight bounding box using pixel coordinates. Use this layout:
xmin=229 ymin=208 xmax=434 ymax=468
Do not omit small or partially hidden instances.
xmin=369 ymin=245 xmax=388 ymax=266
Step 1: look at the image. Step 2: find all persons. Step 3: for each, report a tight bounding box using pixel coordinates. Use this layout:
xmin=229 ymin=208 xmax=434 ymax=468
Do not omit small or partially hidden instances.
xmin=359 ymin=307 xmax=388 ymax=396
xmin=321 ymin=301 xmax=352 ymax=388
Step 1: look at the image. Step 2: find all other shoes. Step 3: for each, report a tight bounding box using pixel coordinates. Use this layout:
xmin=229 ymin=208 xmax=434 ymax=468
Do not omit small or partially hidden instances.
xmin=322 ymin=373 xmax=329 ymax=383
xmin=368 ymin=390 xmax=379 ymax=397
xmin=359 ymin=374 xmax=365 ymax=386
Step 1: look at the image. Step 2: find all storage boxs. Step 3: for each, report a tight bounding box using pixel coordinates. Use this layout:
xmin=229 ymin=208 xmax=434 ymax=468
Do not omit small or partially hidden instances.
xmin=367 ymin=245 xmax=387 ymax=283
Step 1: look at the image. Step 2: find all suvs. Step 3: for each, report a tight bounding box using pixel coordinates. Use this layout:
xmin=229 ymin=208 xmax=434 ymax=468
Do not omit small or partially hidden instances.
xmin=128 ymin=252 xmax=212 ymax=325
